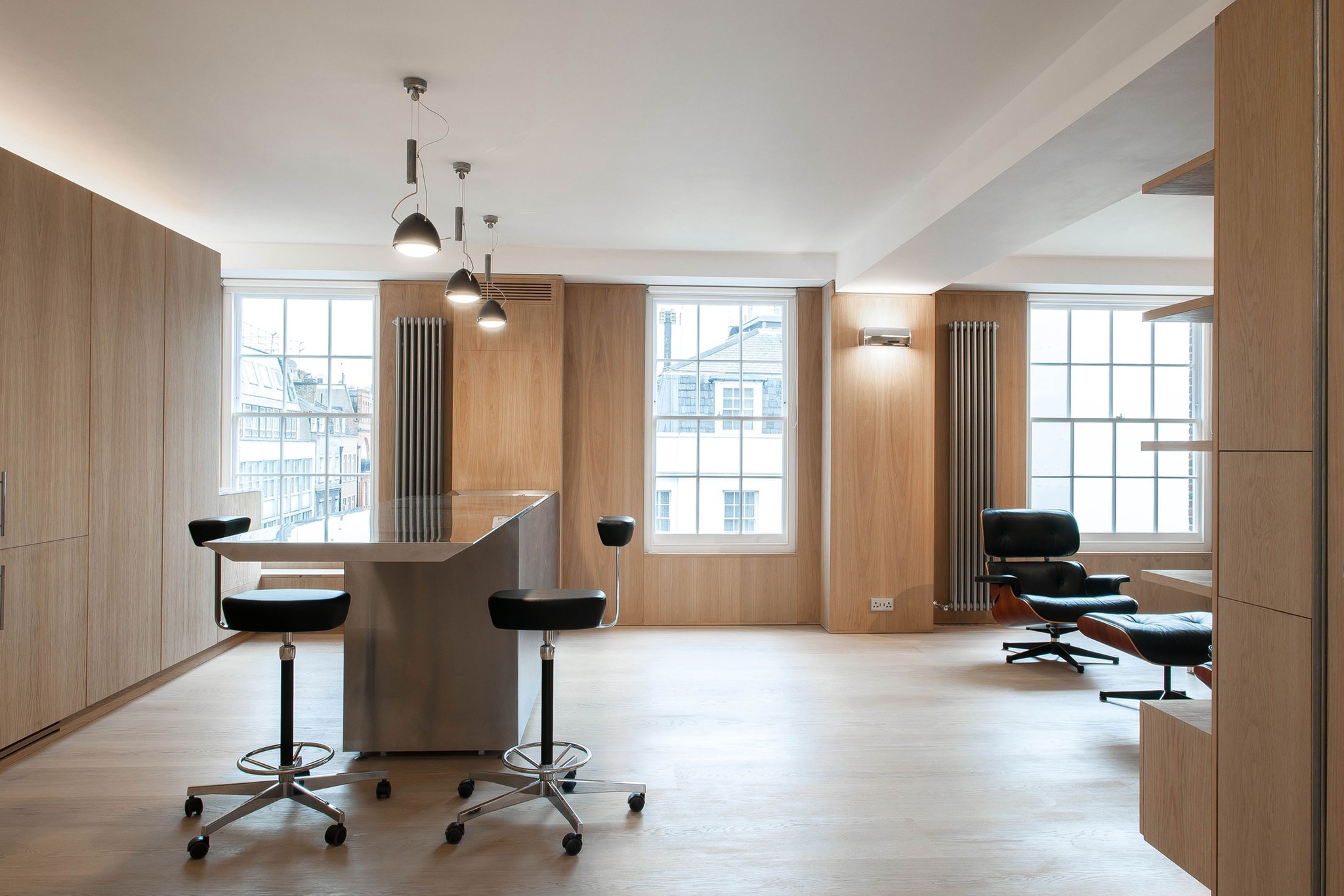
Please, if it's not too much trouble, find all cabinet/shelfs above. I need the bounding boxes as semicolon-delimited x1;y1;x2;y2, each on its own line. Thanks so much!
0;147;261;749
1140;149;1215;890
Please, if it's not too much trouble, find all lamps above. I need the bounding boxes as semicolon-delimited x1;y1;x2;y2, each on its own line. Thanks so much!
859;327;911;347
392;76;507;327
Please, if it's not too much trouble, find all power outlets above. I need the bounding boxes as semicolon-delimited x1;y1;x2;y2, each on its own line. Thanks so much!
870;598;894;611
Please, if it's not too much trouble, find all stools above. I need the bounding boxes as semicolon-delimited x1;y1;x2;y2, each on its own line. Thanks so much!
444;516;647;856
184;516;392;860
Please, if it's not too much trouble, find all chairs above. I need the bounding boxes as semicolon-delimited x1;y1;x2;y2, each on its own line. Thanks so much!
1077;611;1212;700
973;507;1140;674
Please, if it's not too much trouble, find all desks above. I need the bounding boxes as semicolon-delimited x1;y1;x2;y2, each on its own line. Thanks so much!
202;490;560;762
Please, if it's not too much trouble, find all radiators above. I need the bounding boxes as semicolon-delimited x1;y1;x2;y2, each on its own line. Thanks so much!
934;320;999;612
392;317;448;499
